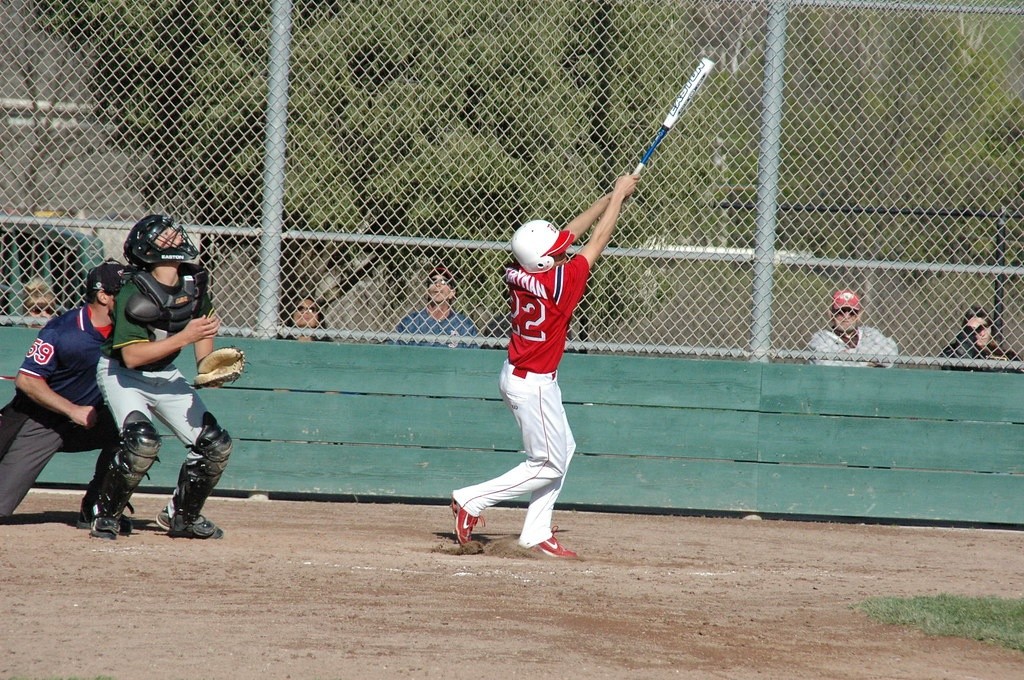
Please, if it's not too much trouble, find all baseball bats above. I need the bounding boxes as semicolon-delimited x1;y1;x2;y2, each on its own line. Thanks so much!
632;56;716;177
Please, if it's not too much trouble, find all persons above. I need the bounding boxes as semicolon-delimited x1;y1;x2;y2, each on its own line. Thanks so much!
452;174;641;557
21;277;55;328
940;308;1021;374
0;256;133;535
90;214;245;540
391;268;478;348
807;289;898;369
482;262;578;352
278;296;334;342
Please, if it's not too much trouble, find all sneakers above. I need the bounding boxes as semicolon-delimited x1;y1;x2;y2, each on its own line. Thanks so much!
452;499;486;545
528;526;576;558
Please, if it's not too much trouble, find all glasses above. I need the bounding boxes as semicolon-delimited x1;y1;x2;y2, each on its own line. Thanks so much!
30;305;55;315
296;307;316;313
836;309;858;316
428;278;449;286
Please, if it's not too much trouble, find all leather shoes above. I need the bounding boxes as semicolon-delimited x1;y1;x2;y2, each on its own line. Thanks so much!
512;368;557;380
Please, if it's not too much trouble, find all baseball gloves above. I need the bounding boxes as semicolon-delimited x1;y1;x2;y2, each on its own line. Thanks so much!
193;343;252;389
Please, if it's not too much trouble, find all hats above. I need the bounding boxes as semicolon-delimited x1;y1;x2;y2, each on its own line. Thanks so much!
832;290;860;310
552;234;575;256
427;268;454;288
86;263;123;294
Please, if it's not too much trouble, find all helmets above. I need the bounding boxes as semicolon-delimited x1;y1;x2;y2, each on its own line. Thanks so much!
122;215;199;272
510;219;570;272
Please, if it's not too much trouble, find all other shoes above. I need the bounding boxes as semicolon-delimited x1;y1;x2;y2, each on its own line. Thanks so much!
156;506;172;530
92;502;116;540
169;515;224;539
76;509;133;536
